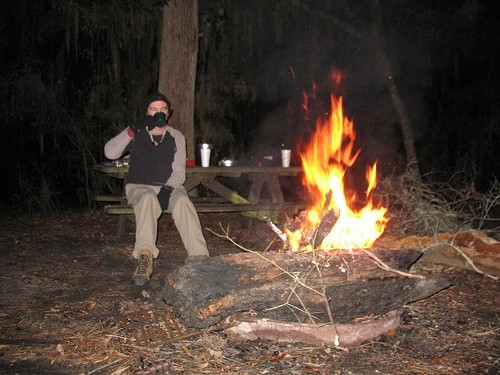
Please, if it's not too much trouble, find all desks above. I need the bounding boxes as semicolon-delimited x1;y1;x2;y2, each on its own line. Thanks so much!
91;164;315;239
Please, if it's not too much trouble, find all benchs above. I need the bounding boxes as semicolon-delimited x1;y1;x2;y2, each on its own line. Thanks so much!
96;196;309;238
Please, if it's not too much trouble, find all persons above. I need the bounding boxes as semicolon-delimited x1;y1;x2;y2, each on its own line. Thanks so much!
104;92;209;282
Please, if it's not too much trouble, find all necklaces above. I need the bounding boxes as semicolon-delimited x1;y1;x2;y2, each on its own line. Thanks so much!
145;125;169;150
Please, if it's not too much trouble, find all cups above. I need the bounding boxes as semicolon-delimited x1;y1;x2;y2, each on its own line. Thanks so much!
281;150;291;168
201;149;211;168
154;112;166;127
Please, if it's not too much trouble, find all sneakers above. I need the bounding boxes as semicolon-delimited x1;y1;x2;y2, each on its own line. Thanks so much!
131;248;153;282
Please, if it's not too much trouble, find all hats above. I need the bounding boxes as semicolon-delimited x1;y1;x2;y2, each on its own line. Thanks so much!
144;92;172;115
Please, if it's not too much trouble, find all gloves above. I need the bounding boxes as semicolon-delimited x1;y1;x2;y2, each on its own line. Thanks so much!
157;186;172;211
130;115;155;133
